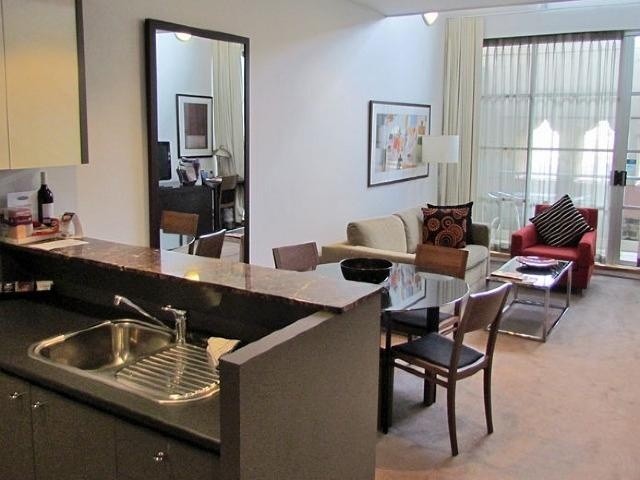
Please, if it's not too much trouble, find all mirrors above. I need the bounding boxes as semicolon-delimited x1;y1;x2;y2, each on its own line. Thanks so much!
144;17;250;261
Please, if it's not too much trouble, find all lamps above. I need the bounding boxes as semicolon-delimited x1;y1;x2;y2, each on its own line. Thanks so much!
421;133;461;205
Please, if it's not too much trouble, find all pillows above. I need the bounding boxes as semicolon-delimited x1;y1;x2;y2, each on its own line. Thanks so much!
420;206;470;248
528;193;594;248
428;201;474;244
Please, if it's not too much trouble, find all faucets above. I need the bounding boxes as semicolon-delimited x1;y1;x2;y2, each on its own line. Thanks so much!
112;294;189;344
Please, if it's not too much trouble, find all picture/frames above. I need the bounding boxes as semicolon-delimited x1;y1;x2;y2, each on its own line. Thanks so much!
367;99;433;188
176;93;214;159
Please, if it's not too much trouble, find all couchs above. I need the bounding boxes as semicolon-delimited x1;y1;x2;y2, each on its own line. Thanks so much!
510;204;600;295
322;201;491;285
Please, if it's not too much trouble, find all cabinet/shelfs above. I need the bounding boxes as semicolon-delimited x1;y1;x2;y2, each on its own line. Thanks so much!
1;0;88;172
0;373;118;479
118;419;221;479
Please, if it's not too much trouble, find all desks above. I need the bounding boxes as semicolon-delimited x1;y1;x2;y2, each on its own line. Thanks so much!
160;231;196;252
310;260;470;435
203;177;244;231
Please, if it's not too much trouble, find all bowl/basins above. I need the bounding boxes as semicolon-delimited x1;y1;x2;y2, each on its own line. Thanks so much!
381;294;393;308
339;257;393;283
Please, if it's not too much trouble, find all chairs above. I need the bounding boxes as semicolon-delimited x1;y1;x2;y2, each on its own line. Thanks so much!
271;239;318;271
161;209;198;255
215;175;238;229
381;244;470;406
383;280;513;458
195;228;227;259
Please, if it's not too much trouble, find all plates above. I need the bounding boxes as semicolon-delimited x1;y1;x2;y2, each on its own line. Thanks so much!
516;255;558;270
515;264;556;276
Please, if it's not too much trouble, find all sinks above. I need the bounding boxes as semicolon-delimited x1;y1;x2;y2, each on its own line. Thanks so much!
28;318;178;373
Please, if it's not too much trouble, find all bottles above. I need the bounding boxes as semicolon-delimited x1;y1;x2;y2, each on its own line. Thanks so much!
37;170;55;224
